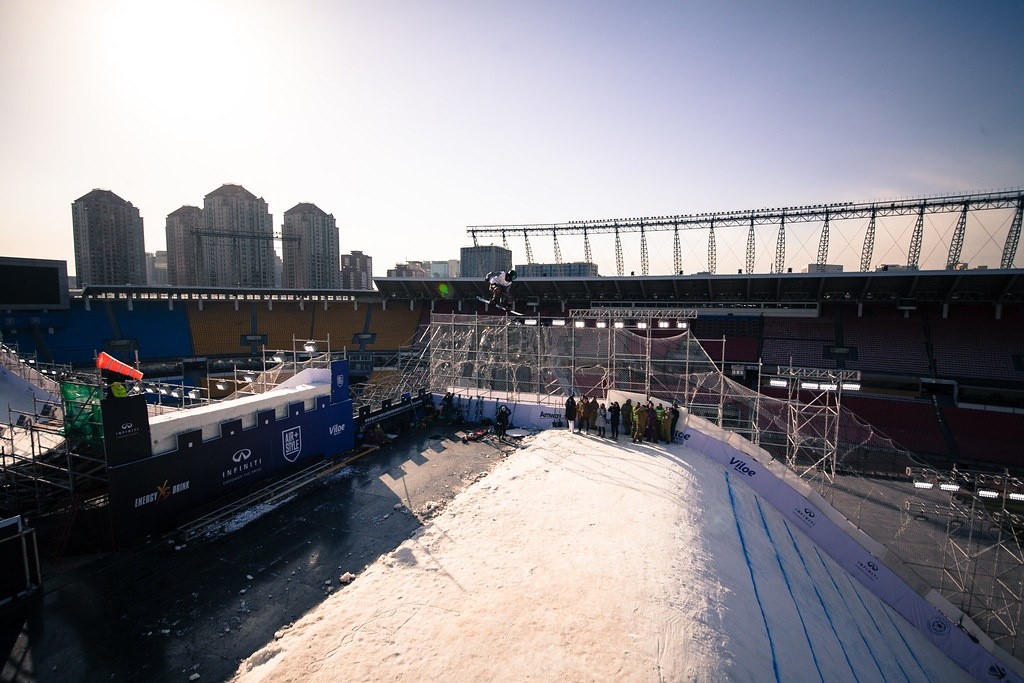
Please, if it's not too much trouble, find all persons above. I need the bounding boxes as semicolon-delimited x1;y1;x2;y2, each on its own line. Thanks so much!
497;406;508;441
486;269;518;313
566;396;680;444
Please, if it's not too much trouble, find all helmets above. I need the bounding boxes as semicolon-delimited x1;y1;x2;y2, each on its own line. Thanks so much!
505;269;518;282
